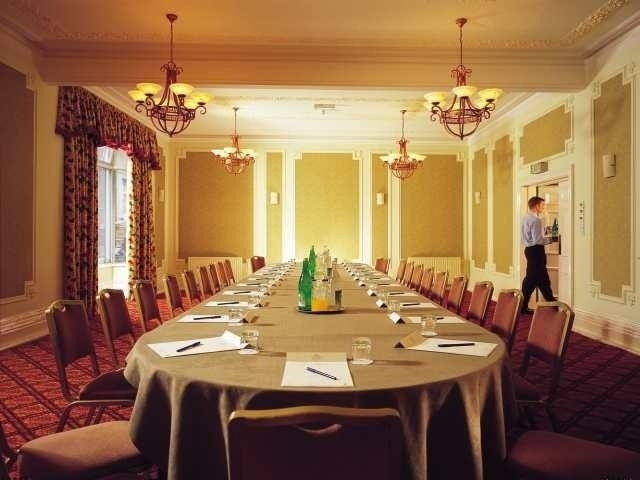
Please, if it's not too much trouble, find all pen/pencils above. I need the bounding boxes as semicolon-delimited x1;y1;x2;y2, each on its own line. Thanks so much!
234;291;251;294
217;301;239;305
193;316;221;320
438;343;475;347
378;284;389;285
437;317;443;320
247;283;259;285
176;342;200;352
403;303;419;306
306;367;336;380
389;293;404;295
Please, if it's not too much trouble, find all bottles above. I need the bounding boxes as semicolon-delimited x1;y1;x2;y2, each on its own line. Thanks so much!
297;245;342;312
551;217;560;241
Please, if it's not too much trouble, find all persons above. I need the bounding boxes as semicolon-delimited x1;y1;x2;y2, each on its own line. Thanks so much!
519;196;558;314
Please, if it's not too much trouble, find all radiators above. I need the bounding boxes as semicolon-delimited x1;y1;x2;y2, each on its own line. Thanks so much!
187;254;243;295
406;255;461;287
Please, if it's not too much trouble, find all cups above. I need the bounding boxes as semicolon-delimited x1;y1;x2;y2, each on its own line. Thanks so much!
227;309;244;326
248;259;296;310
351;336;371;364
239;330;259;354
420;314;438;337
350;265;390;303
387;298;401;314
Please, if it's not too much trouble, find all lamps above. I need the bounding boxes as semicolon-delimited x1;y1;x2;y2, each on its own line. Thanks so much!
212;107;259;177
380;109;429;182
126;12;216;141
422;17;503;139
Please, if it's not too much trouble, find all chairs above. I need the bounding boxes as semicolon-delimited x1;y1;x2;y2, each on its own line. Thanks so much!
0;417;151;479
496;298;579;427
510;428;637;480
133;252;504;480
46;299;135;421
94;288;137;365
131;279;163;334
491;289;522;362
